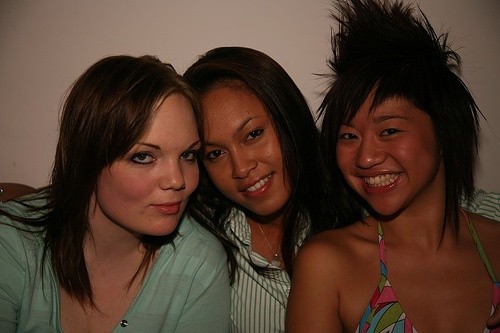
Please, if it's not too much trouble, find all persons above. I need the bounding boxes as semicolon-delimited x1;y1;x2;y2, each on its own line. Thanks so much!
0;46;370;333
284;0;500;333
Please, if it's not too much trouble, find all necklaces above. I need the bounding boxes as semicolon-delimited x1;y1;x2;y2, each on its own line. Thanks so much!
255;218;283;257
